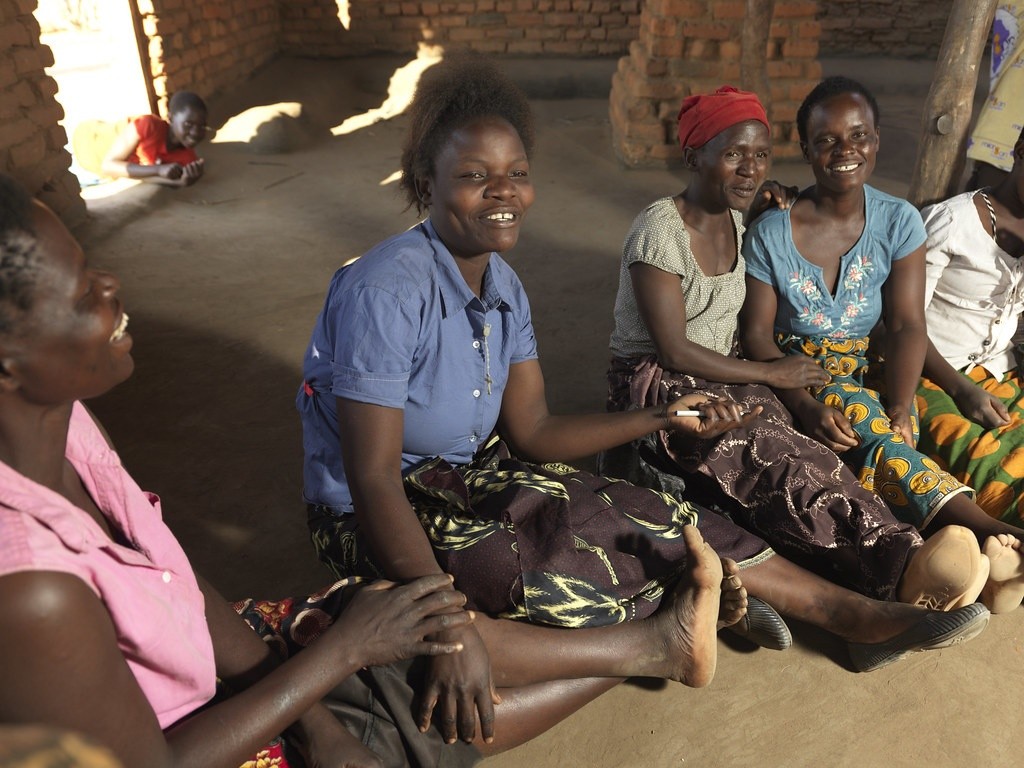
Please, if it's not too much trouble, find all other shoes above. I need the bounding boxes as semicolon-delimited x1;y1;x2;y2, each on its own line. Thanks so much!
847;603;990;672
902;525;981;611
744;594;792;651
940;553;990;612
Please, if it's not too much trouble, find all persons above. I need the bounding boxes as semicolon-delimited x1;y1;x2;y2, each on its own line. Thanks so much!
0;185;748;768
298;57;989;747
608;86;991;644
64;90;208;188
915;127;1024;529
737;78;1024;615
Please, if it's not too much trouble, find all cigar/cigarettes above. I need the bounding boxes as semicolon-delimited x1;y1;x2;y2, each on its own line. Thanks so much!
675;410;748;417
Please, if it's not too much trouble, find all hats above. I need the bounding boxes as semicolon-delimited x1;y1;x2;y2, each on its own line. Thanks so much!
676;85;771;154
